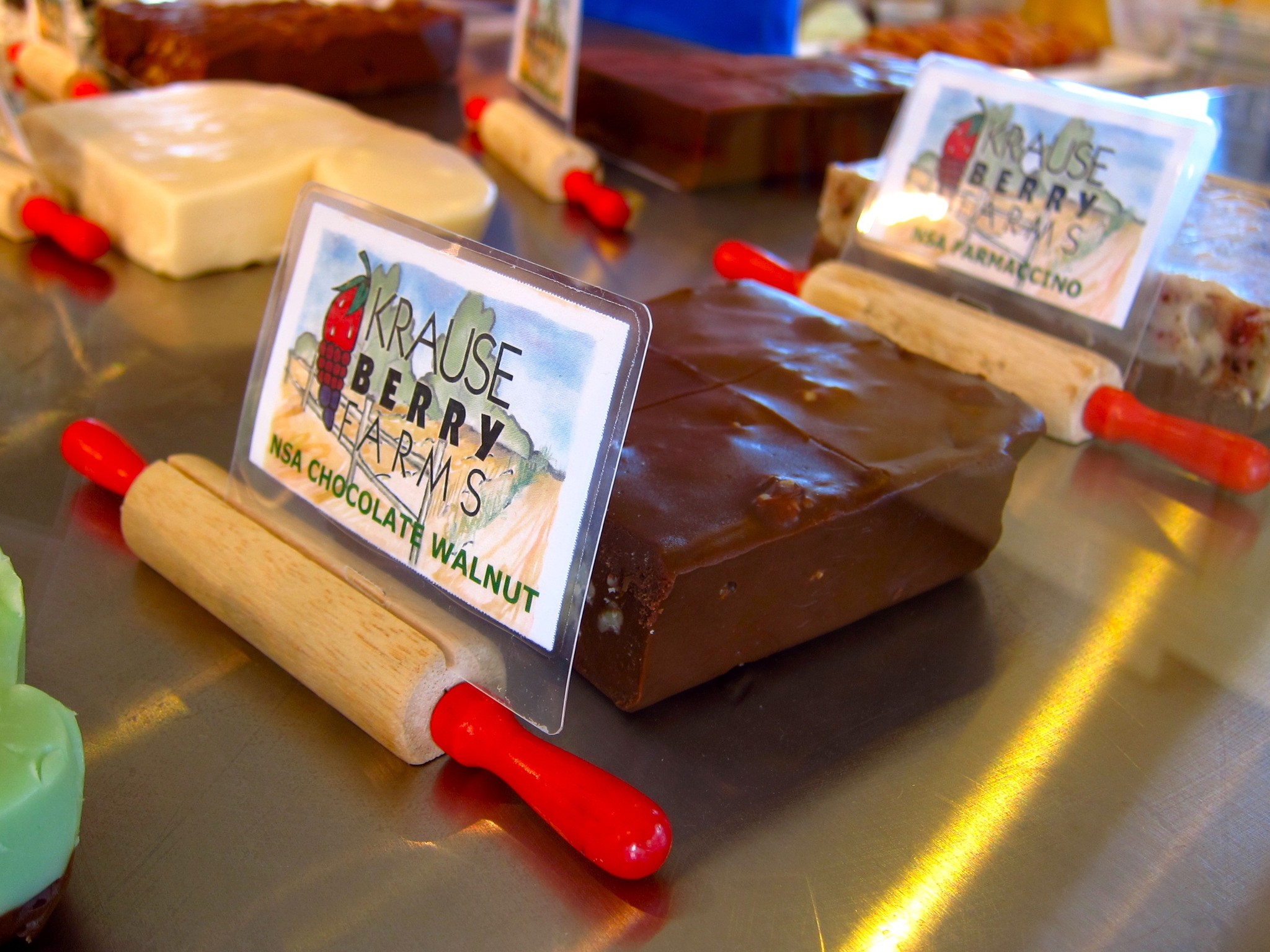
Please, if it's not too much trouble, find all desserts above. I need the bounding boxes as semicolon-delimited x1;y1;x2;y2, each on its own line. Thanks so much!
94;1;464;93
810;158;1268;407
571;277;1048;715
21;81;502;280
575;24;906;192
